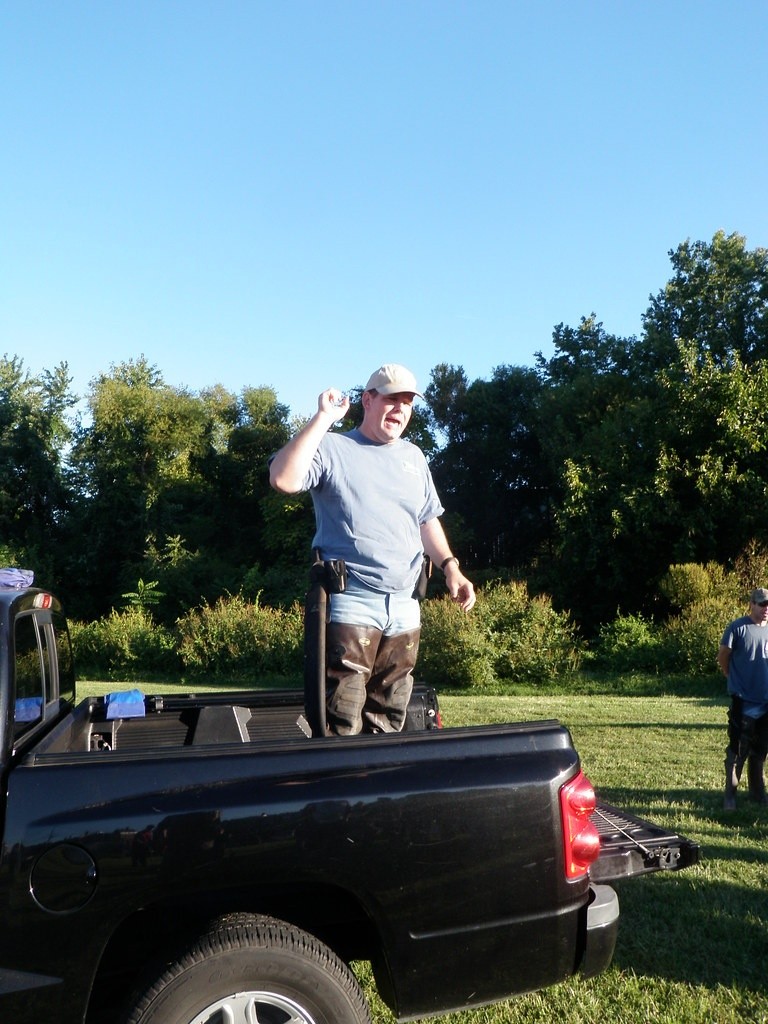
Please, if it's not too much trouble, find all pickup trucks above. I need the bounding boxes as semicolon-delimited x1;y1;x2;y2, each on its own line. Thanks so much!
0;583;701;1024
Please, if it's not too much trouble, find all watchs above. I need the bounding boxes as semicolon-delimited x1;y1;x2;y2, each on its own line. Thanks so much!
440;557;459;572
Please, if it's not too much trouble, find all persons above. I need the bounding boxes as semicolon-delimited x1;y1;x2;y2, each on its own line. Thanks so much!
271;364;477;737
715;587;768;813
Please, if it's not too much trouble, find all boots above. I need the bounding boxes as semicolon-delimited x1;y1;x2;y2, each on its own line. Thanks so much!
325;622;383;737
747;763;768;803
723;760;743;813
362;626;421;733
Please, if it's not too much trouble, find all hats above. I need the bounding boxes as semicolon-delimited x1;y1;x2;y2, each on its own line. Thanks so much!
751;589;768;603
364;363;424;400
